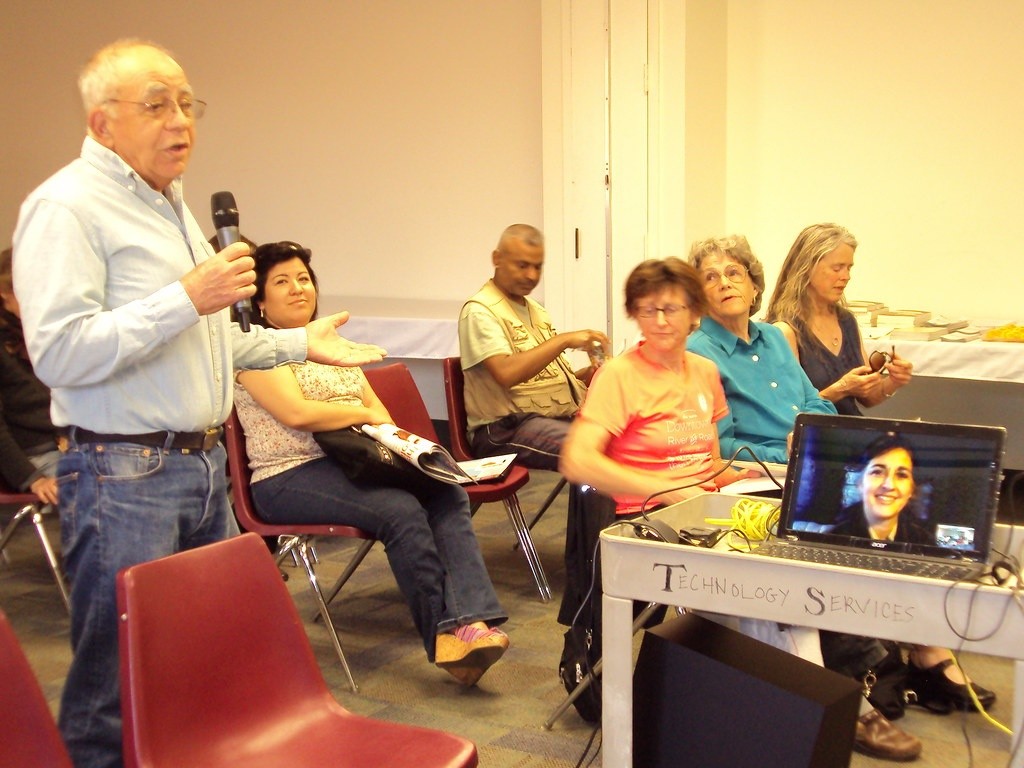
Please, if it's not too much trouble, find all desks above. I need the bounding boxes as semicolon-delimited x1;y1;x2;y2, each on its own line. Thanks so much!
336;315;462;420
861;337;1024;472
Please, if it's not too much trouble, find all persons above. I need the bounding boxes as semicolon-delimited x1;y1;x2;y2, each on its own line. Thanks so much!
234;241;511;687
684;237;952;720
558;258;923;763
765;224;997;714
823;435;935;545
458;224;618;580
208;233;289;581
11;40;387;768
0;249;58;507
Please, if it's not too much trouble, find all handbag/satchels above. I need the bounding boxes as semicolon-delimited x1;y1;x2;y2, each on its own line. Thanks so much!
311;424;425;489
558;625;605;723
830;663;905;720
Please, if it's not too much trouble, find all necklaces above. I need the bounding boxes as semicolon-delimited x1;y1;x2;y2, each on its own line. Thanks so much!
832;338;837;346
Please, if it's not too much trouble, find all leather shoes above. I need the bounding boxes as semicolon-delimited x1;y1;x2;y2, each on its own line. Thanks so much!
907;653;996;705
853;695;922;760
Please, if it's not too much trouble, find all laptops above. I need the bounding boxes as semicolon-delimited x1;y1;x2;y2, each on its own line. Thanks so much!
749;414;1007;584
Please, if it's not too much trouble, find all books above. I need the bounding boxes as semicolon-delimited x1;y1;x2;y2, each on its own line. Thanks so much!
844;300;997;342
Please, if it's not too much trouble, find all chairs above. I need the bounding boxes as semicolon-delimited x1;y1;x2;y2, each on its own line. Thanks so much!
0;356;659;768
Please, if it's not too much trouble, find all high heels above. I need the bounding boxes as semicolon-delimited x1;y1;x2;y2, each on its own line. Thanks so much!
435;625;507;667
446;628;507;686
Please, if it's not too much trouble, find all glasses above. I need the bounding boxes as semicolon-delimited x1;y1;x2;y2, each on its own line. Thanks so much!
701;263;751;288
637;305;697;318
258;241;305;261
865;345;895;374
109;97;207;119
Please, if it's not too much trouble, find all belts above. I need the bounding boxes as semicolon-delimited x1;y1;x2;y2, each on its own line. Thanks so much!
54;424;222;450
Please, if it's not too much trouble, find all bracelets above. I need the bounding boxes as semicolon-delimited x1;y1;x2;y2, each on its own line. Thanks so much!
883;391;897;398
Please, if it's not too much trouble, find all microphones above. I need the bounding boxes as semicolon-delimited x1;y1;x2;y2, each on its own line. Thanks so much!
211;190;254;333
